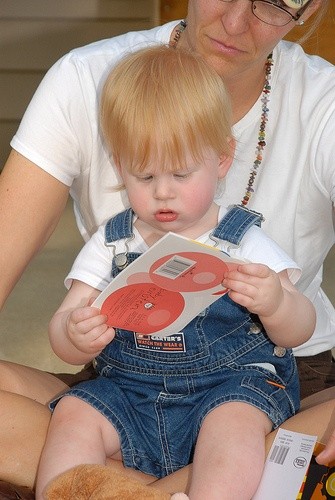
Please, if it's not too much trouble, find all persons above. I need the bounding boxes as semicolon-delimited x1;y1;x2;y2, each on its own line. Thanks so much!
0;0;335;500
35;45;317;500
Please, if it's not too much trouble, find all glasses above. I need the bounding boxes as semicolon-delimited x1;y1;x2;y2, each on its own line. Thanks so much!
250;0;315;28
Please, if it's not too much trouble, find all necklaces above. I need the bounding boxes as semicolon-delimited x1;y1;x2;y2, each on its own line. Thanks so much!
172;20;275;209
